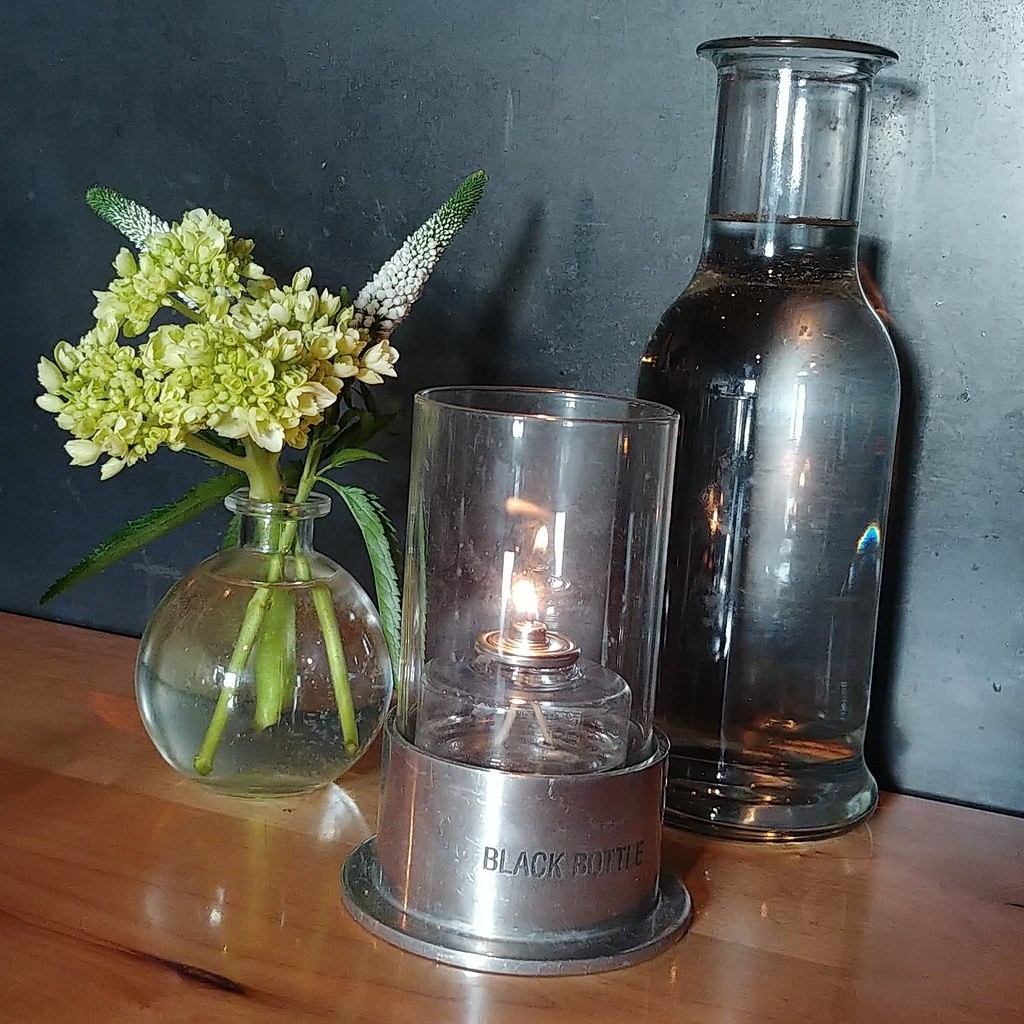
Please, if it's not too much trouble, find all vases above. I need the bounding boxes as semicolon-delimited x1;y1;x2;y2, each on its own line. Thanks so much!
134;489;395;799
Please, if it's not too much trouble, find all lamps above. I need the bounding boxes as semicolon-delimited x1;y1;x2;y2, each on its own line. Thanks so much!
341;386;693;974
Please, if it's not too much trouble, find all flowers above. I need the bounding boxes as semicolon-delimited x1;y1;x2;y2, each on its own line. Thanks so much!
39;173;489;775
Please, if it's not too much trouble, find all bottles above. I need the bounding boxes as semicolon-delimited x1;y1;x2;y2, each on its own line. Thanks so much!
639;36;904;842
